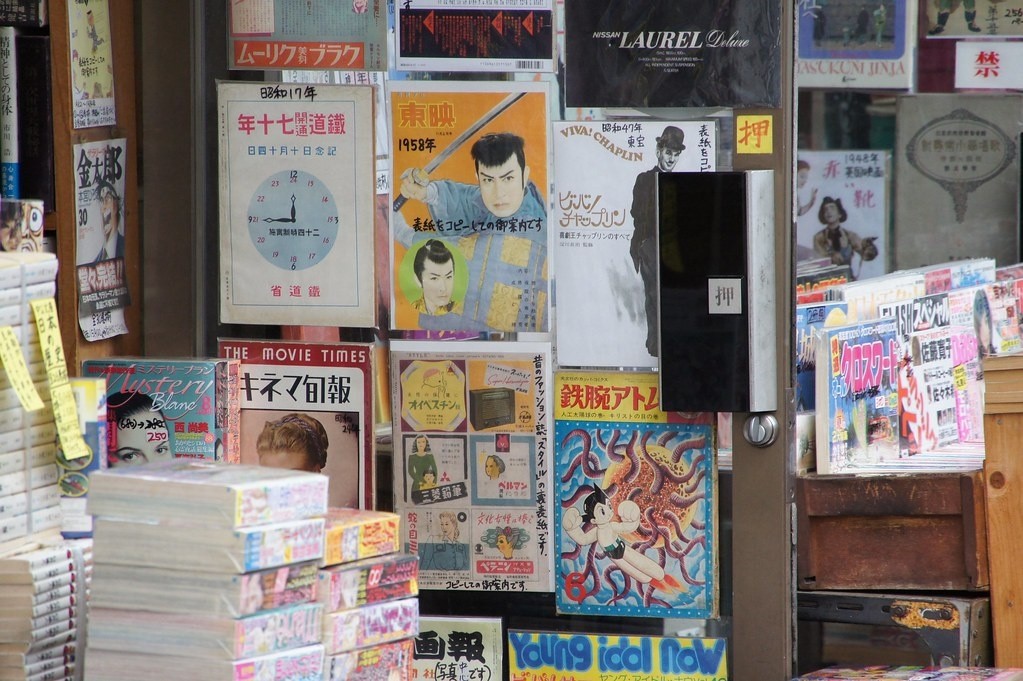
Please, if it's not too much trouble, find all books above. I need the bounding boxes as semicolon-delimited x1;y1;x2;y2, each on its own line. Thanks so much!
383;0;778;681
58;342;419;681
0;0;91;681
794;254;1022;477
794;0;1020;282
219;76;379;330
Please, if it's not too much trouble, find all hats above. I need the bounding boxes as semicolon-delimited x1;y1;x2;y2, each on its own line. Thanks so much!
655;126;685;150
